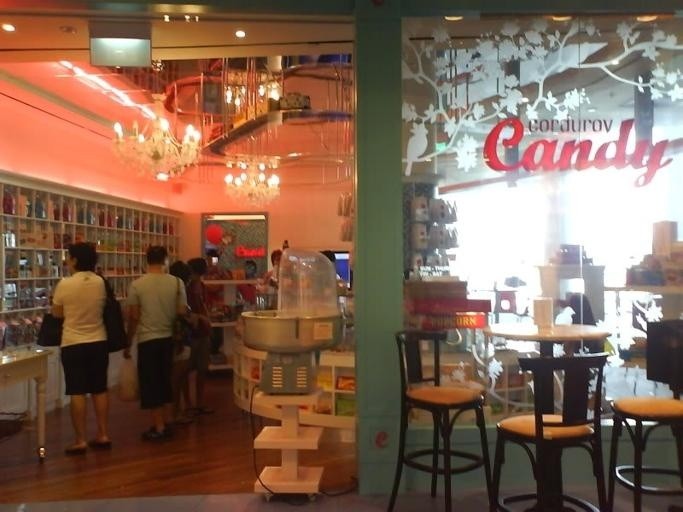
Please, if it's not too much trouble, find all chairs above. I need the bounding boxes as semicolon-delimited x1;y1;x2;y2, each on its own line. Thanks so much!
490;352;606;512
389;330;494;509
608;320;682;511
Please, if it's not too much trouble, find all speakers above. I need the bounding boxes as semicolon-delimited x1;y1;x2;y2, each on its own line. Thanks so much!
87;22;153;69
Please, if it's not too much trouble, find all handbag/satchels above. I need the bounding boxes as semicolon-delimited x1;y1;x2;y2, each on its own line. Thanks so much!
172;321;192;374
38;313;64;347
97;274;129;353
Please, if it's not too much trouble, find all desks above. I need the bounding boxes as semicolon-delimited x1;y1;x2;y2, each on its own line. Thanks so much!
1;347;52;460
484;323;612;418
537;260;604;329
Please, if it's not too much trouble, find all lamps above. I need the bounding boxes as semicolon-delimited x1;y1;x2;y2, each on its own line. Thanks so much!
111;61;200;178
224;52;279;105
224;139;281;204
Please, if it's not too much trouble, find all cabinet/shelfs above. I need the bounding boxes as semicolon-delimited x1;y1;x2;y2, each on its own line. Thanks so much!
0;344;60;421
61;337;136;406
610;223;682;371
250;387;325;501
0;168;184;346
232;345;529;428
198;275;264;370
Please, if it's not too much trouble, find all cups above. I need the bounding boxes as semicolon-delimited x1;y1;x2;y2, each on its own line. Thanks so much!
537;326;553;335
533;298;553;328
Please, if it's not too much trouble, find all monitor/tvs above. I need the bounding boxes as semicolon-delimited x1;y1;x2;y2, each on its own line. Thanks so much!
332;251;352;291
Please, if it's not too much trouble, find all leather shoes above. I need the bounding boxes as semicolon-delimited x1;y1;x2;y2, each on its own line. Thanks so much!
66;443;87;453
88;436;112;449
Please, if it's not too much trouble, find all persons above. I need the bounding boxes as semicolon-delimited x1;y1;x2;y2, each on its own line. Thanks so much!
259;250;298;310
237;258;259;306
50;240;115;455
118;247;192;442
167;259;200;428
185;256;226;416
203;244;229;316
310;249;351;306
